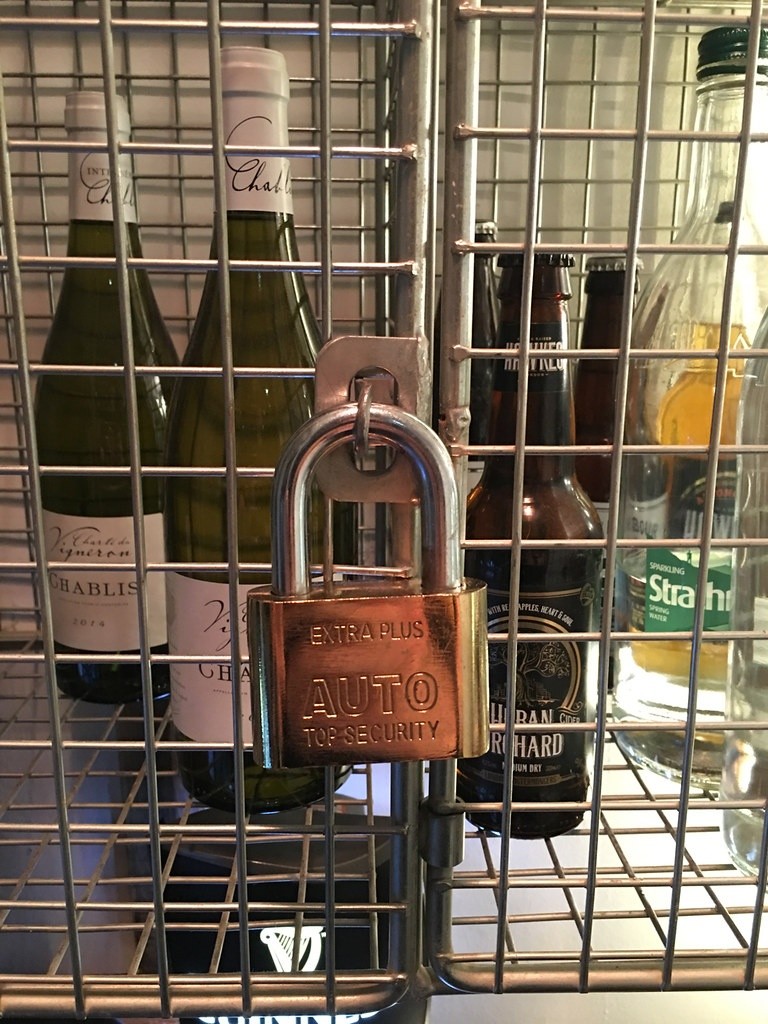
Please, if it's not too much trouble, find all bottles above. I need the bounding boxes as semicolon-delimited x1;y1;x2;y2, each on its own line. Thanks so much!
34;92;181;700
163;45;360;804
436;204;768;880
610;25;768;791
